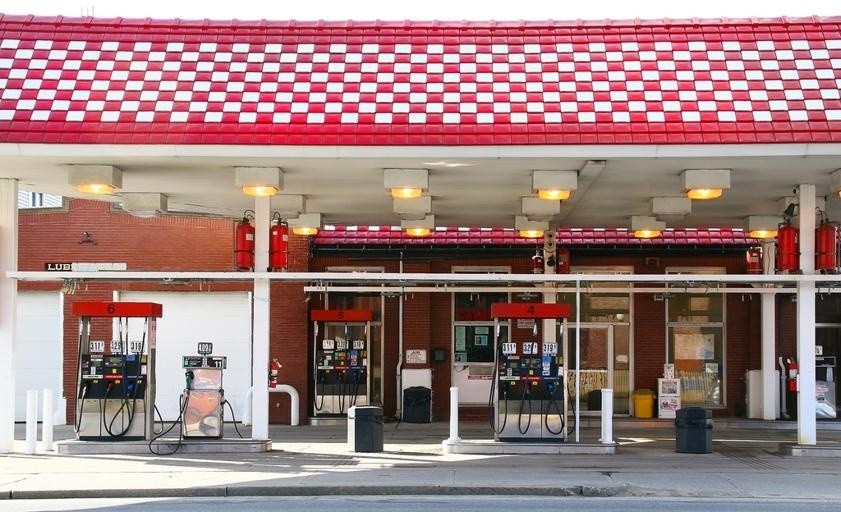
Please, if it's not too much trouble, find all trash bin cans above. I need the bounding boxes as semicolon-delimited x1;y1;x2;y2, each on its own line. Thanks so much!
674;407;713;454
588;390;601;410
403;386;431;423
632;388;657;419
347;405;384;453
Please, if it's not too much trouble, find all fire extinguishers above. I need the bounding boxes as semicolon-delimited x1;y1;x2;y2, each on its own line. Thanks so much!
556;246;569;274
269;358;283;388
814;206;837;271
746;243;764;274
269;211;289;272
778;204;798;272
233;209;255;271
789;356;798;392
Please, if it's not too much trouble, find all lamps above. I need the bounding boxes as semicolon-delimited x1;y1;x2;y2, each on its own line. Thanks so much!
648;197;692;221
521;196;561;221
744;215;778;238
401;215;435;236
384;168;429;199
629;216;666;238
234;168;284;196
531;170;577;199
393;196;431;220
680;170;731;199
121;193;167;218
271;196;306;219
514;215;549;237
66;164;123;196
288;213;323;234
